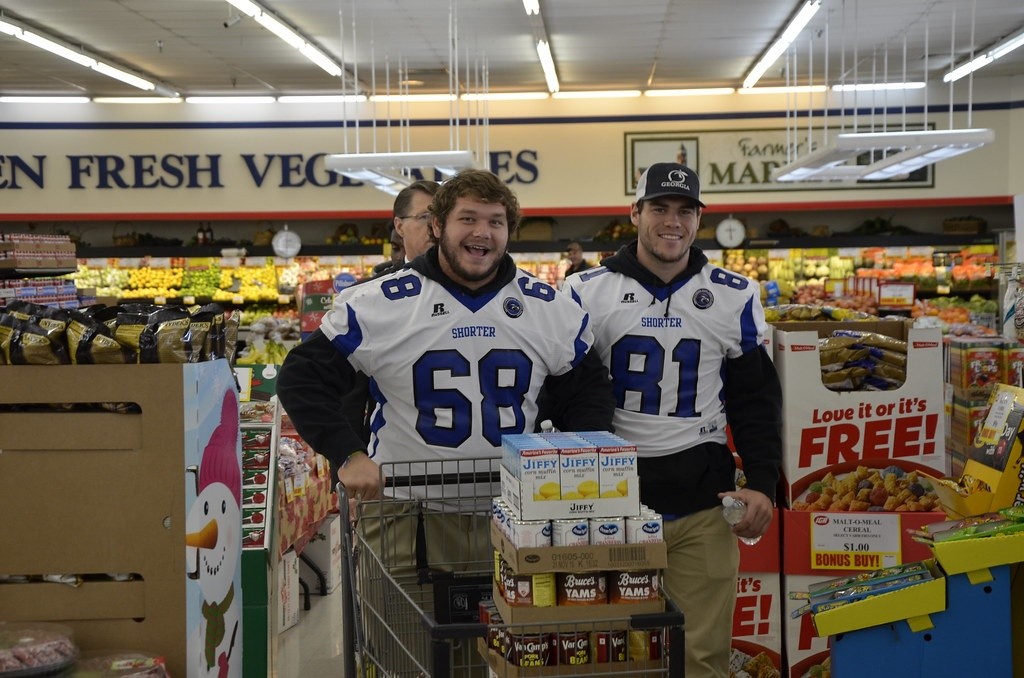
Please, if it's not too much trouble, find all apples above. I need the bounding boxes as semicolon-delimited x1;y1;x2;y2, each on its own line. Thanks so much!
238;302;299;326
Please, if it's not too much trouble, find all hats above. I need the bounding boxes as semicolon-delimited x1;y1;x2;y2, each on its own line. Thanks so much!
565;242;581;252
637;163;706;208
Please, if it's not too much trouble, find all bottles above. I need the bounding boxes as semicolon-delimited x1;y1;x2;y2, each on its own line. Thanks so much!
722;495;761;545
540;420;562;433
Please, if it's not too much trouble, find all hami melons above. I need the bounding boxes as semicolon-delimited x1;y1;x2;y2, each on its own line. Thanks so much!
724;255;768;280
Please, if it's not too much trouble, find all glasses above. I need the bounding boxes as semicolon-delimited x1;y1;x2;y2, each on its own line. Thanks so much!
397;212;436;223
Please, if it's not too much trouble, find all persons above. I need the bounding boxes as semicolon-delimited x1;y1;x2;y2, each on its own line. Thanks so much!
371;222;407;278
275;169;617;678
329;180;440;522
565;243;596;276
563;163;783;678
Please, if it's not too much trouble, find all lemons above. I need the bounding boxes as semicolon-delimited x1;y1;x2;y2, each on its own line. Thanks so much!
119;268;280;302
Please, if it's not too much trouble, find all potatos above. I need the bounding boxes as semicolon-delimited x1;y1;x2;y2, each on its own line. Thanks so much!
792;285;876;315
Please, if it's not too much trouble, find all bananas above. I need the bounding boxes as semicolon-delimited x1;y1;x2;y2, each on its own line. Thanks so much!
236;337;300;365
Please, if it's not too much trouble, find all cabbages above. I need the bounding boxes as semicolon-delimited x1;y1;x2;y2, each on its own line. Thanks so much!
769;261;831;287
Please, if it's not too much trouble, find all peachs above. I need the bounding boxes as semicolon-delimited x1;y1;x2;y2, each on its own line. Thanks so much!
521;264;556;289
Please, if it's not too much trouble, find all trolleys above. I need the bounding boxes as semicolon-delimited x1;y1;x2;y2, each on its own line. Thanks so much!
336;456;687;678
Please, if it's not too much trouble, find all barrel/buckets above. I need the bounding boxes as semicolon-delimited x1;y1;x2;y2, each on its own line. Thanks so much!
481;496;664;668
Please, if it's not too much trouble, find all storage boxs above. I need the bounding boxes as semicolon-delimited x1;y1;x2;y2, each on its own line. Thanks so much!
476;464;666;677
0;241;96;309
0;359;242;678
772;320;1024;678
277;509;343;632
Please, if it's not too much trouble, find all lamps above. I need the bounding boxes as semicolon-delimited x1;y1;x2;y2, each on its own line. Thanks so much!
772;0;994;181
325;0;490;196
0;0;1024;103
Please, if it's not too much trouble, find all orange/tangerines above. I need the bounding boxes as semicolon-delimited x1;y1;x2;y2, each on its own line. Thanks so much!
912;303;993;334
